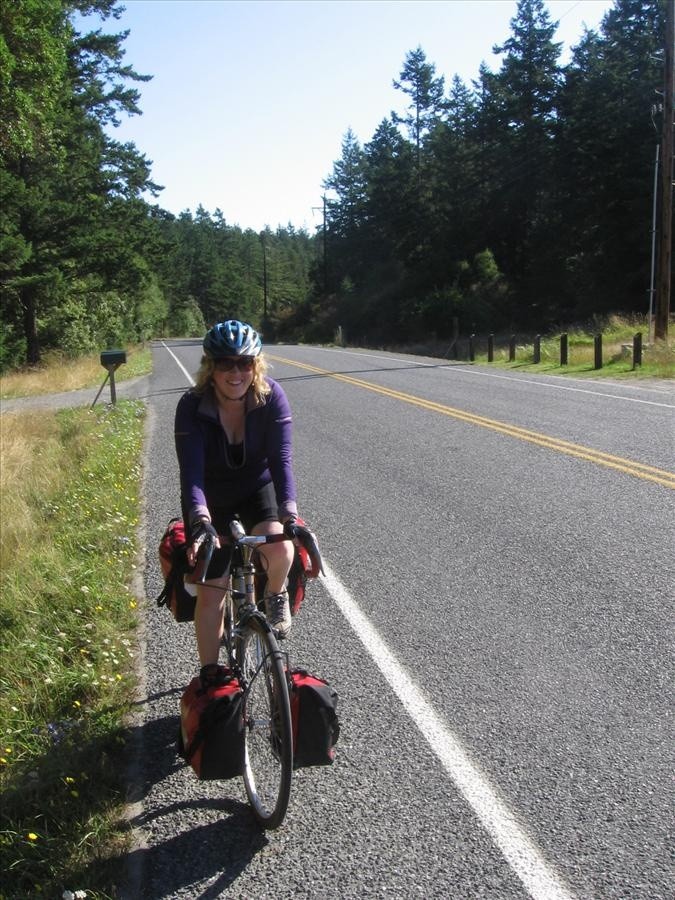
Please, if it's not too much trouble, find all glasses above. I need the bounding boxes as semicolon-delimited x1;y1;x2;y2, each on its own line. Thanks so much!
213;357;256;371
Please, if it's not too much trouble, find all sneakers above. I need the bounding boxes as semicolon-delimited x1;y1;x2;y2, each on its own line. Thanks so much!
263;580;291;638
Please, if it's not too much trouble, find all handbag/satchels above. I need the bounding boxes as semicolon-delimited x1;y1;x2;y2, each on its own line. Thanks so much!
157;517;198;623
254;516;320;614
176;664;245;780
272;666;340;771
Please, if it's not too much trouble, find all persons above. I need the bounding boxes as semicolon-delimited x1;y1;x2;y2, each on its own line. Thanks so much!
174;321;311;691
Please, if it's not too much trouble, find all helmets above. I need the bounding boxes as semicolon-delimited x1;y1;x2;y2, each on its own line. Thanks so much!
203;320;263;357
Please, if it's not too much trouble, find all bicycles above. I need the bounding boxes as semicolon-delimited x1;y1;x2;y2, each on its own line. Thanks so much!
194;514;328;831
